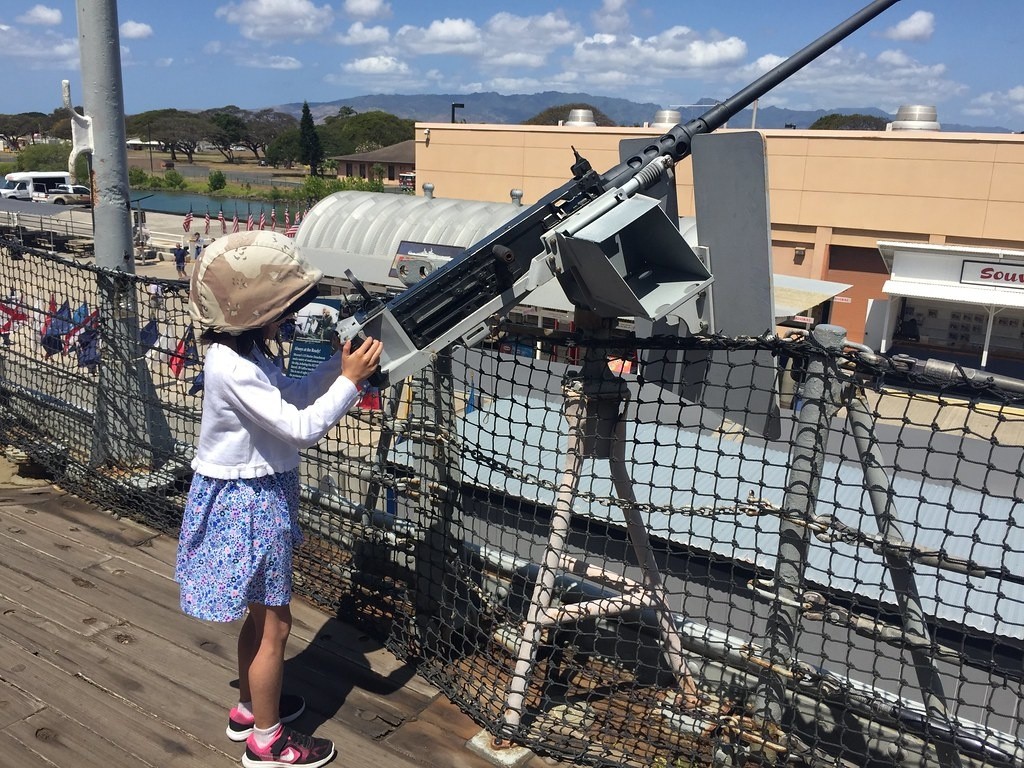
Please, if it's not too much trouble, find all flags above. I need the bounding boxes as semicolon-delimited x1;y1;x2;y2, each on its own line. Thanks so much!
204;204;211;234
232;207;240;233
217;203;227;234
183;201;193;232
245;197;310;238
0;288;200;380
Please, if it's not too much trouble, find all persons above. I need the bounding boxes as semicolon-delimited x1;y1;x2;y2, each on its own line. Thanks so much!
173;231;384;768
896;317;920;342
149;233;205;320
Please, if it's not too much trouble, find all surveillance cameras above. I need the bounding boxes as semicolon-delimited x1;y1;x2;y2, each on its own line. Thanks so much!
424;130;428;135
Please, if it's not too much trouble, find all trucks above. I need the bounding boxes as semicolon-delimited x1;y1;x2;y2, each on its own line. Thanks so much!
0;171;71;201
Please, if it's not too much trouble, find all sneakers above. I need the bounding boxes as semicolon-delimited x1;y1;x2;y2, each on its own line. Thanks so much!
225;692;306;742
242;724;335;768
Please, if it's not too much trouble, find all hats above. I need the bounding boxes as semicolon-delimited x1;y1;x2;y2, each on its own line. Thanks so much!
188;230;323;334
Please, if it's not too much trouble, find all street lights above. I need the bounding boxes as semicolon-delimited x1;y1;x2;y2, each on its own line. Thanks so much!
148;120;154;171
452;102;465;123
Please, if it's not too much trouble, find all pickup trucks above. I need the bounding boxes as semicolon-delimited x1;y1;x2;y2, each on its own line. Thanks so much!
31;180;92;205
